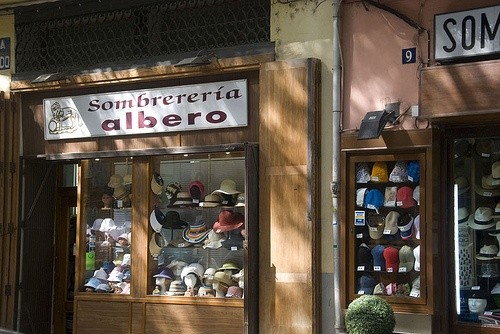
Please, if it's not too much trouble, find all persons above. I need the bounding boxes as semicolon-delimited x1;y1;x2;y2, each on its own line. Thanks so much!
468;290;487;314
187;181;204;207
100;190;113;209
108;175;130;201
165;182;182;208
211;180;241;205
117;234;128;246
152;262;244;299
85;270;128;294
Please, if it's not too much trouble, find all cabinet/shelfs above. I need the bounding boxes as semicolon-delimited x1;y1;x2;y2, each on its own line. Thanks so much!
448;137;500;328
72;150;258;334
341;127;438;314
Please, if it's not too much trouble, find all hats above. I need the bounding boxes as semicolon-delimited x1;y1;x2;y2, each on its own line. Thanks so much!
450;141;499;299
355;161;419;298
83;172;245;299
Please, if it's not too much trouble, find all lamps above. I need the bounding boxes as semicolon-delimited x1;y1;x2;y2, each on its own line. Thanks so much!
357;99;402;141
